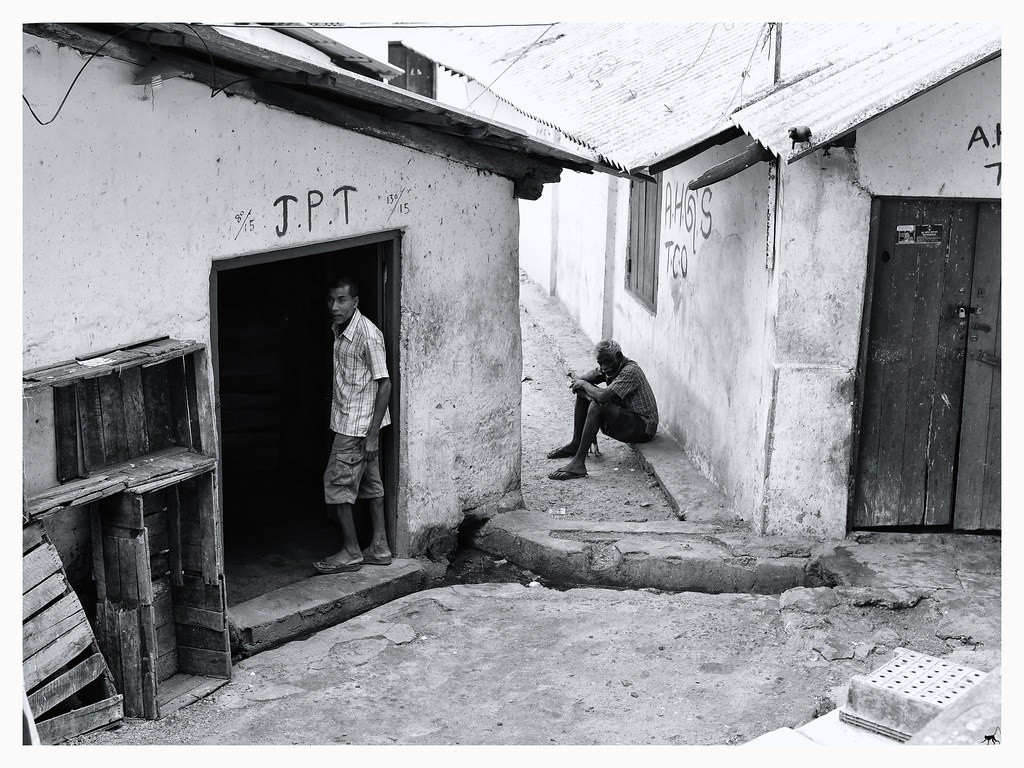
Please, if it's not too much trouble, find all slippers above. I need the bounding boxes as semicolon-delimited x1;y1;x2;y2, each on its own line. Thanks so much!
547;448;577;459
314;555;360;574
362;550;392;565
549;468;587;479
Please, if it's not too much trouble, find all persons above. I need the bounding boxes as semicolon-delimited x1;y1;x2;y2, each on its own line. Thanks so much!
546;339;659;480
310;277;392;573
